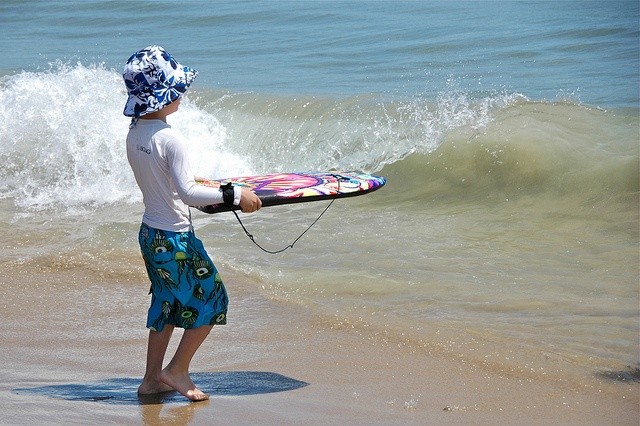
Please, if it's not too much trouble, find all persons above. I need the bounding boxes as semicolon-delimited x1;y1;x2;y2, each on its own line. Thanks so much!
120;43;265;403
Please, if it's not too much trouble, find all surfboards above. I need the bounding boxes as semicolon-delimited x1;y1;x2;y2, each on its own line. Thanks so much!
193;172;386;214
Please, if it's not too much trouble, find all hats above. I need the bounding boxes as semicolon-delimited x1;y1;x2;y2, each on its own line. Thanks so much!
122;44;199;129
